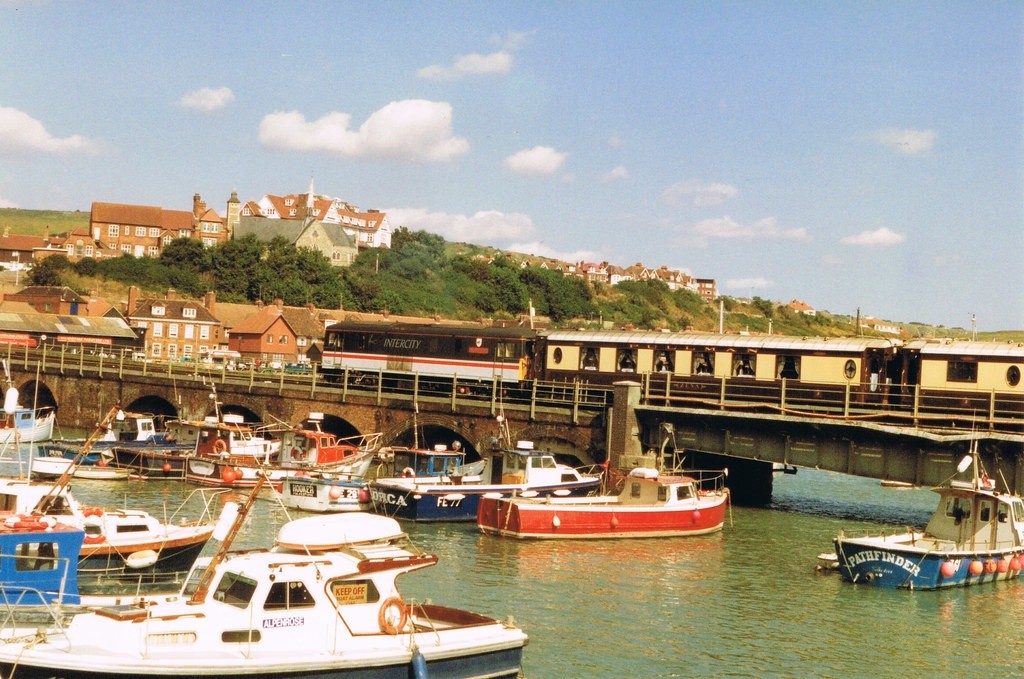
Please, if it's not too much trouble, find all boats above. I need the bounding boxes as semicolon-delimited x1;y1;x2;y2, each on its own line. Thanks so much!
0;404;233;586
0;359;55;443
832;408;1024;590
280;474;378;511
37;433;176;464
31;456;135;480
477;423;733;540
368;344;604;521
0;360;119;518
112;439;281;479
0;477;528;679
185;359;383;488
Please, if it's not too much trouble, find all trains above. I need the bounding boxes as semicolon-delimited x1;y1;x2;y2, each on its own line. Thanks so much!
321;314;1024;431
